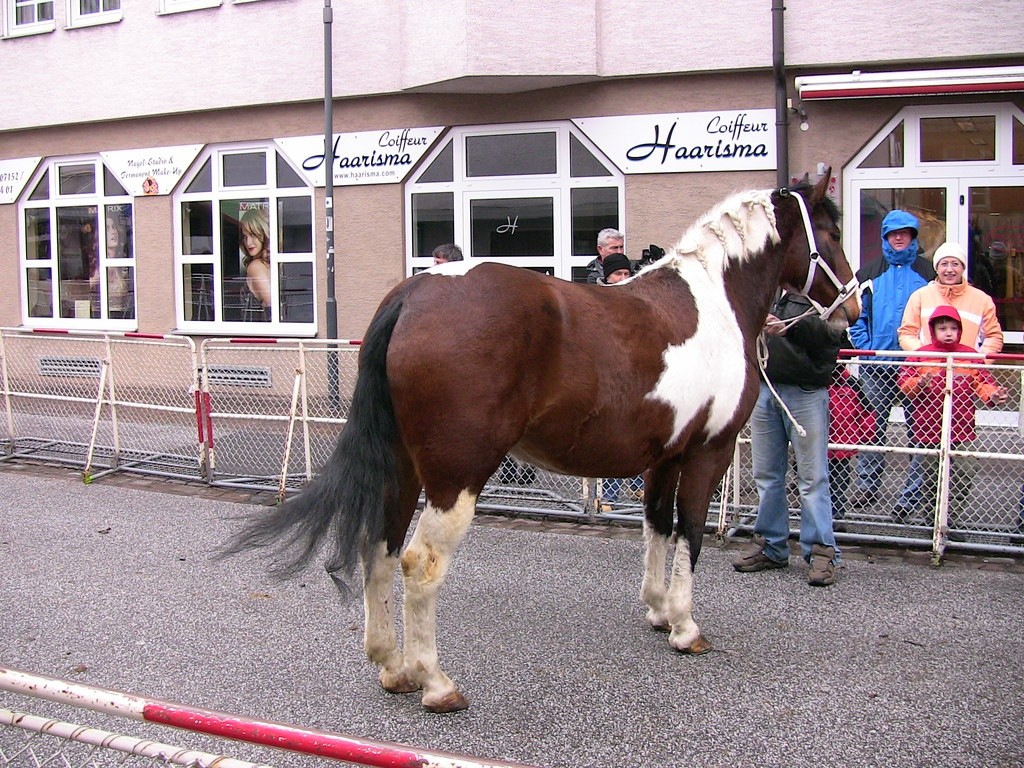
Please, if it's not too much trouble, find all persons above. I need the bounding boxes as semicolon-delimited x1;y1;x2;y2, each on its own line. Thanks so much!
500;456;536;483
431;243;464;267
826;331;876;533
595;253;633;286
891;307;1008;542
586;228;661;510
89;213;129;311
893;242;1003;517
848;209;937;516
238;209;271;322
732;284;842;587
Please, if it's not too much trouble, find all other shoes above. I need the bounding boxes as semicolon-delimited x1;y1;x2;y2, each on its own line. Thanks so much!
1010;518;1024;546
849;489;878;508
929;522;965;542
891;504;912;520
594;497;613;511
629;488;645;501
832;517;848;532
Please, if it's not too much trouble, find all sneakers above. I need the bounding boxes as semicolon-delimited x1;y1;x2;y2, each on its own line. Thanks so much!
807;544;835;586
733;532;789;572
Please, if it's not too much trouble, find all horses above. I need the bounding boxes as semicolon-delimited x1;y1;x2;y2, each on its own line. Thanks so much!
205;165;861;713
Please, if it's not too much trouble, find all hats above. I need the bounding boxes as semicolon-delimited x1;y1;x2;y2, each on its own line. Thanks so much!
990;241;1008;260
601;252;632;282
933;242;967;271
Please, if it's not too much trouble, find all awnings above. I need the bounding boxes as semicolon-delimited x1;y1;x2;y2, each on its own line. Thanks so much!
793;65;1024;100
